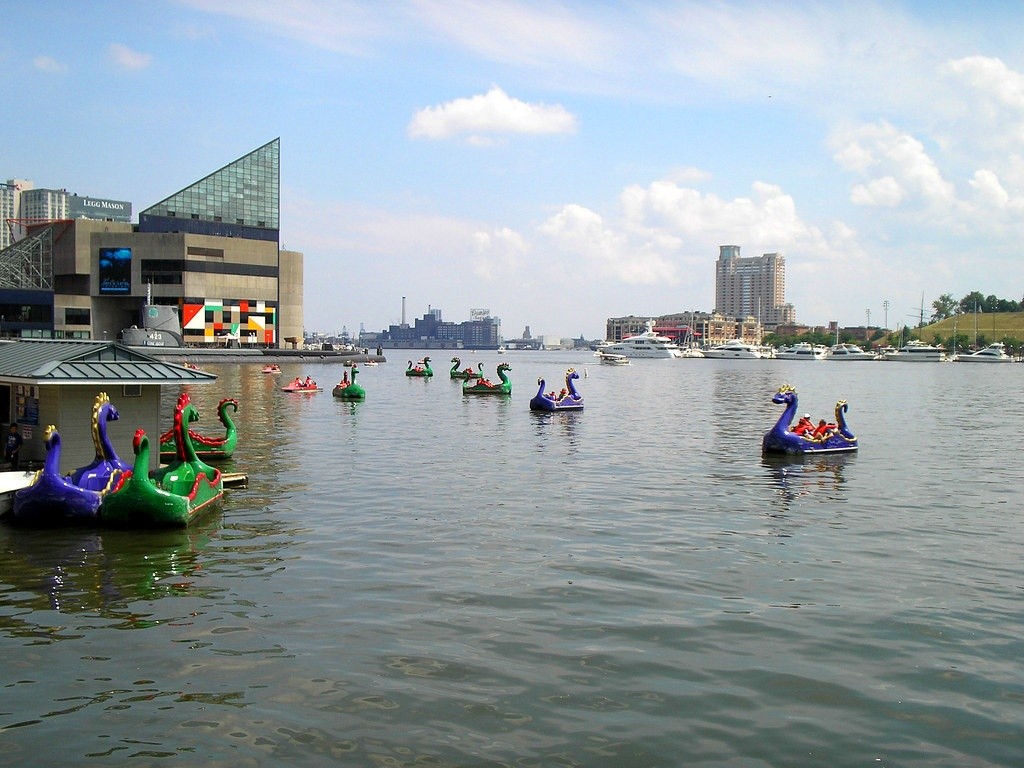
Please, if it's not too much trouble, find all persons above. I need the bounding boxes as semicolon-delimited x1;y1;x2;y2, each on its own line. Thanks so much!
549;387;567;401
4;423;23;471
478;377;493;388
794;413;838;444
266;363;473;389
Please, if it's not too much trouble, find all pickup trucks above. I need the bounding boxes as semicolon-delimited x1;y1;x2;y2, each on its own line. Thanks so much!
813;344;829;355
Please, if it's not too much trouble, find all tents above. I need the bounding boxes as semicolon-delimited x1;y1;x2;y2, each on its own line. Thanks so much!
217;333;241;349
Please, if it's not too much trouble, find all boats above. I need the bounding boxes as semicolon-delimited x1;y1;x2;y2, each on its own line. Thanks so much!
98;391;224;528
343;360;352;366
183;363;199;371
599;353;630;366
884;339;947;363
450;357;485;378
160;398;239;462
599;332;682;359
496;346;505;354
762;383;859;456
364;361;378;366
700;340;764;359
405;356;434;377
530;367;585;412
462;362;512;396
12;391;134;527
954;342;1016;363
332;362;366;400
822;343;878;360
774;343;816;360
281;379;321;392
262;365;282;375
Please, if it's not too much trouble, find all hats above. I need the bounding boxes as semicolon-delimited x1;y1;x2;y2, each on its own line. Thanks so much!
804;413;810;418
